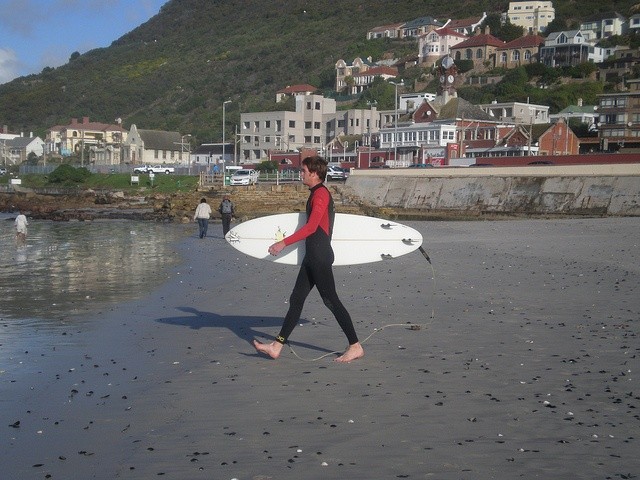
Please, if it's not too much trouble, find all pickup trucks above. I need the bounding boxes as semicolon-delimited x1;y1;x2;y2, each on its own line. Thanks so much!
148;164;175;175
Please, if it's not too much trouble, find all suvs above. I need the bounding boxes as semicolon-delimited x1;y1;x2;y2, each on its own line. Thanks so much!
134;164;151;173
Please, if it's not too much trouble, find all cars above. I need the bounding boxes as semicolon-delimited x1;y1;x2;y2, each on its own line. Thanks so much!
0;168;7;176
528;160;554;166
325;166;349;181
469;163;496;168
369;165;390;169
408;164;432;168
230;168;258;186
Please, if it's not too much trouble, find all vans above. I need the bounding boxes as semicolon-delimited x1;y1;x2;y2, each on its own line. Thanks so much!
225;166;243;181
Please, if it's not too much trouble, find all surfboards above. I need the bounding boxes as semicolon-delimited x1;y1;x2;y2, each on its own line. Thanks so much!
225;212;424;266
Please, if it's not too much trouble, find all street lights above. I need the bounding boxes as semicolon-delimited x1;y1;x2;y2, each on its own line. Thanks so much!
389;81;405;168
180;134;192;167
222;100;234;187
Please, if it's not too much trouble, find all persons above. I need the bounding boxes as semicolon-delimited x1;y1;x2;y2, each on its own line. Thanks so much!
193;198;212;239
14;210;30;239
218;195;234;239
252;156;364;363
